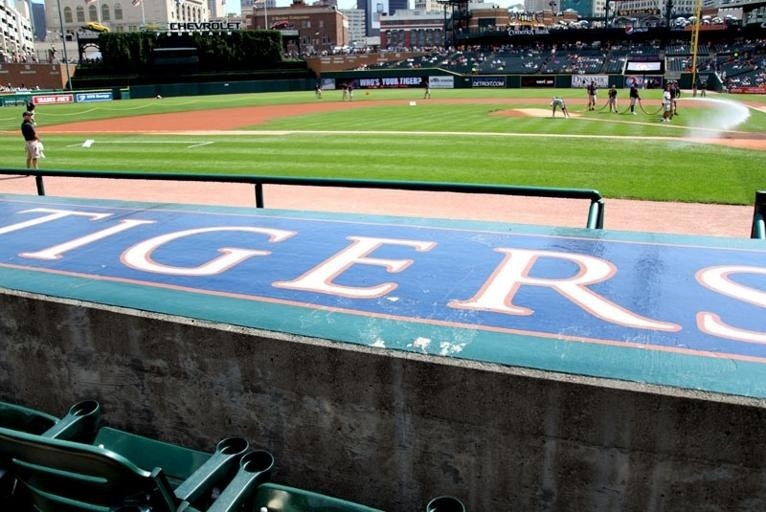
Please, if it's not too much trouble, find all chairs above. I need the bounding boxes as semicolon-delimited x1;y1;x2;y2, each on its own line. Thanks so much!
0;399;251;511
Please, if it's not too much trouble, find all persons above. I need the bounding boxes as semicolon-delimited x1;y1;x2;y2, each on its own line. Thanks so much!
21;102;45;169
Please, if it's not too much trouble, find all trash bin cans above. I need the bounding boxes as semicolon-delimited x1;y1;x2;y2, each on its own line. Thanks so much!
119;88;130;99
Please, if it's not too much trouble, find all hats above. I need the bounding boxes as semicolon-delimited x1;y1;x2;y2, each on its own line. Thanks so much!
22;111;32;116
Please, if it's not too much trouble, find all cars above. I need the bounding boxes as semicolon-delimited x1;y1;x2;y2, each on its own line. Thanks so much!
80;21;111;34
268;20;288;31
